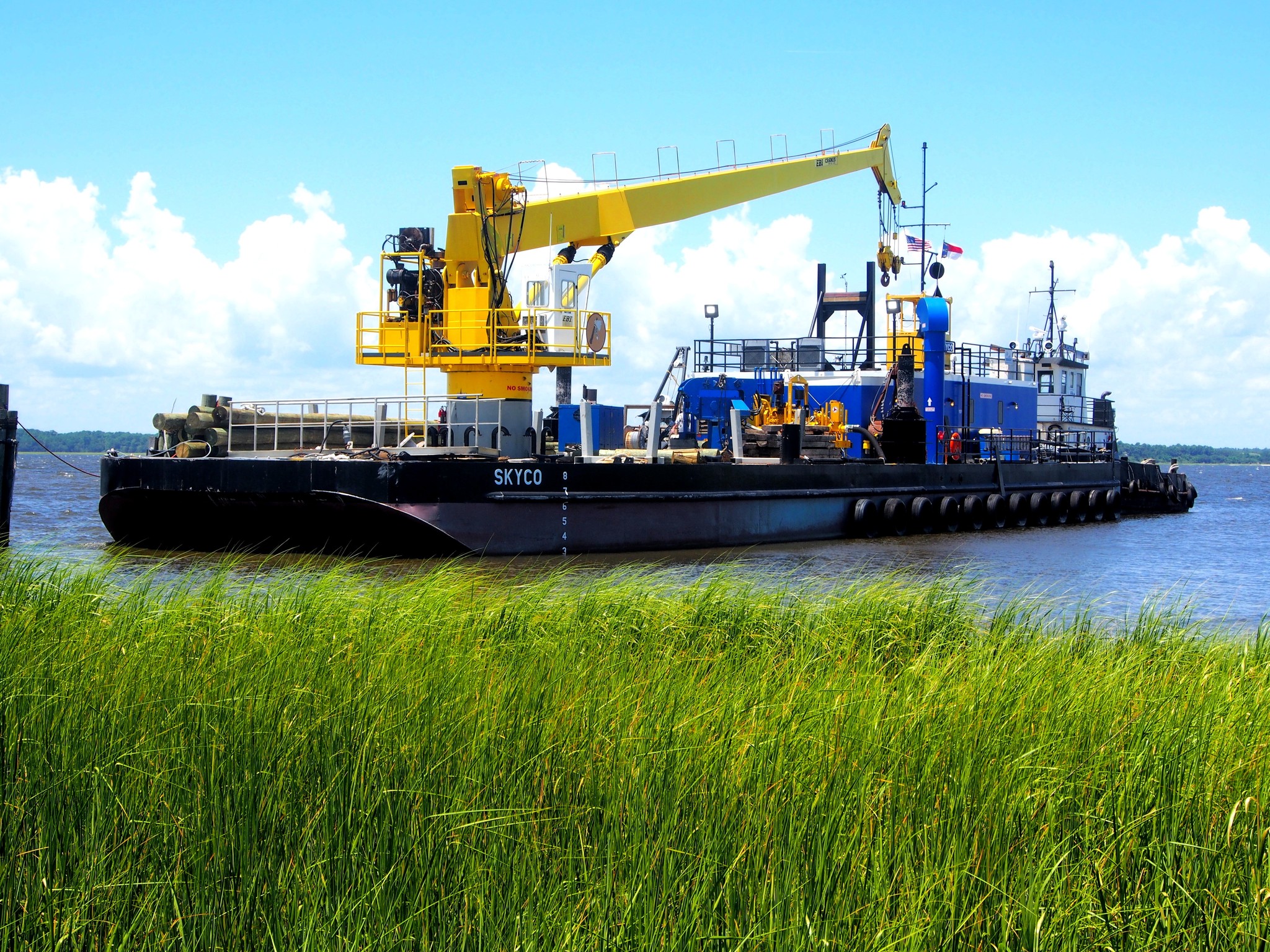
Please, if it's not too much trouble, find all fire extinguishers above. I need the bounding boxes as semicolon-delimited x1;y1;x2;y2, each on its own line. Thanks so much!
437;405;447;434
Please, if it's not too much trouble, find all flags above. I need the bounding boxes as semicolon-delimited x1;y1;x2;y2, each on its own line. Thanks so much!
906;235;932;251
941;242;963;260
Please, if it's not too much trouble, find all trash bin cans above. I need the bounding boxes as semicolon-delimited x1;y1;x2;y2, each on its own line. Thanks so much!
779;424;800;464
1121;457;1129;461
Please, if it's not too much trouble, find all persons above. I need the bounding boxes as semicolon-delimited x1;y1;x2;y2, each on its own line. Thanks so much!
672;413;691;432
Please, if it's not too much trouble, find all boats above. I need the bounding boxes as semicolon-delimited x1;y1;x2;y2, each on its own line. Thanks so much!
99;125;1202;560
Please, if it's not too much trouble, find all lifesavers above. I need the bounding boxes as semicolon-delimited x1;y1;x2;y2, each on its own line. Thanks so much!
1070;490;1089;523
963;494;985;532
1187;483;1198;508
939;496;961;534
911;496;934;534
854;497;878;538
1009;492;1030;527
986;493;1008;529
1049;491;1069;526
1159;483;1169;504
1089;489;1106;522
1168;484;1181;504
1030;491;1049;528
883;497;908;537
1128;480;1140;499
1106;489;1123;521
950;433;961;460
